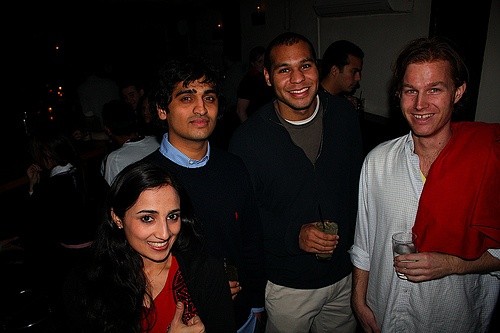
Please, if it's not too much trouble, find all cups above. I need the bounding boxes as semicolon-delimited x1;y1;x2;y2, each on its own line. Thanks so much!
313;221;339;260
392;232;418;279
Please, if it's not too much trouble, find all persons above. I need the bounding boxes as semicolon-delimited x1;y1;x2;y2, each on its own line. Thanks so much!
82;164;237;333
231;33;384;333
237;46;273;123
318;40;365;113
91;77;165;187
133;53;265;333
348;37;500;333
0;142;102;272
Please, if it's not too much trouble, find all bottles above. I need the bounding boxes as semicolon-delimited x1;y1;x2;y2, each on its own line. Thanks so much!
356;96;365;114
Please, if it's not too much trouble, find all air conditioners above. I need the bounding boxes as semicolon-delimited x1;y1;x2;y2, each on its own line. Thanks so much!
312;0;414;18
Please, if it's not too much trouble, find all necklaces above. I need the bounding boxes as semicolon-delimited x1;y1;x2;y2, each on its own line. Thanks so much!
146;260;169;288
